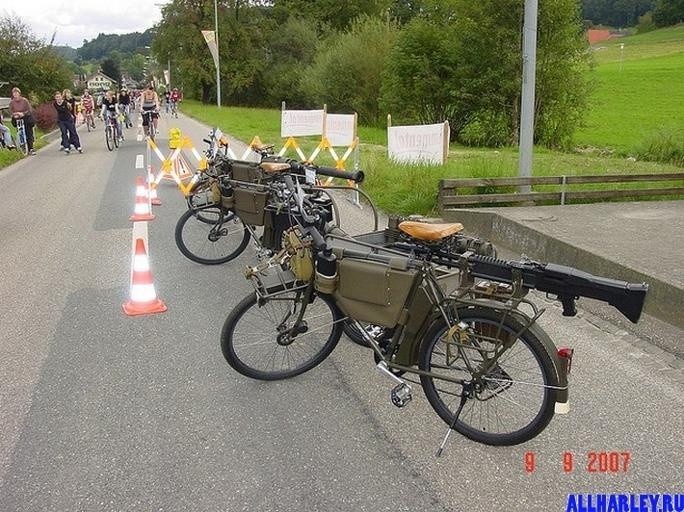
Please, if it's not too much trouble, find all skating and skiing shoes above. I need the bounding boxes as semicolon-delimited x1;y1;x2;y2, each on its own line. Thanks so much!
59;145;82;154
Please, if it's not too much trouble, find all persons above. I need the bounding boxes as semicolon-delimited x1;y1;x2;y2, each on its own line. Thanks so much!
53;83;183;154
0;114;15;148
9;87;36;155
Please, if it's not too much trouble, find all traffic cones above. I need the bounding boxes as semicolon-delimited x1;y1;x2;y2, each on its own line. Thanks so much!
122;165;167;316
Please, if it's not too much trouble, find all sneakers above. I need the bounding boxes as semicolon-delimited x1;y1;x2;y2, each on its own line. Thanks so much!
29;149;36;155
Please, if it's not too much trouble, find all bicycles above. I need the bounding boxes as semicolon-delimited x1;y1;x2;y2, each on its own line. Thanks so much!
174;128;574;457
11;114;37;157
81;99;183;151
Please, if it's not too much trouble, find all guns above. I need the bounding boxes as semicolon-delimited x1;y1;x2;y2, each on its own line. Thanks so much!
385;240;649;322
260;149;365;184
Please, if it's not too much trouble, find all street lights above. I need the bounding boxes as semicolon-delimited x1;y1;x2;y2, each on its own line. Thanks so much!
142;30;171;88
